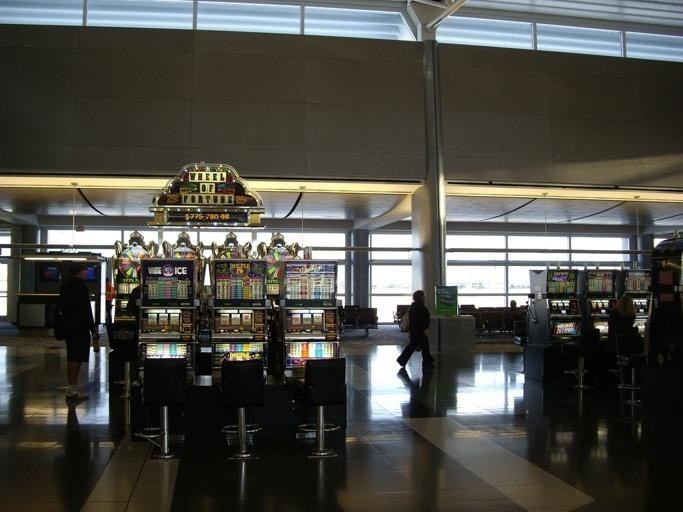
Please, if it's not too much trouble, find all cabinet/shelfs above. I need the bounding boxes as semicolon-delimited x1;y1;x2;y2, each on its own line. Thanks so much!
524;269;652;416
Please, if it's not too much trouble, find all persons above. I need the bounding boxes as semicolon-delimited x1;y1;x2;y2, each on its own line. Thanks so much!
126;270;142;316
62;264;99;402
106;276;116;322
397;289;437;370
505;301;522;321
608;295;637;350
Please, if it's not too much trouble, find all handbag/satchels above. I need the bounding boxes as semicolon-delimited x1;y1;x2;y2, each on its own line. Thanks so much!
399;314;409;331
52;310;66;340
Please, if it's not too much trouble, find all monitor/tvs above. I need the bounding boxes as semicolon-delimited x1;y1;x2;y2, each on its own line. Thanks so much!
41;264;61;281
80;265;98;281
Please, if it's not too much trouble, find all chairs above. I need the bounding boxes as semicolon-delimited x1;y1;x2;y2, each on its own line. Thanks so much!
338;305;378;337
135;359;347;460
394;305;530;334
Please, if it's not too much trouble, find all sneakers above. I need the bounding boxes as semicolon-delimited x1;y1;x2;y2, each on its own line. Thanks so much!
65;389;88;398
422;362;434;368
